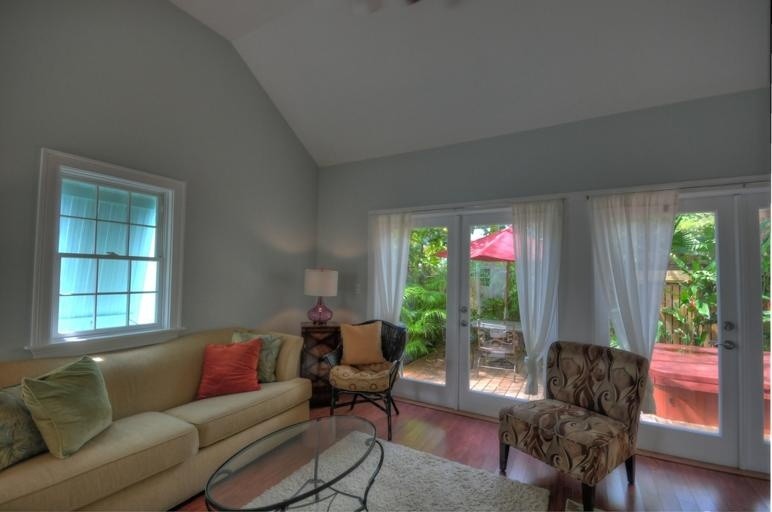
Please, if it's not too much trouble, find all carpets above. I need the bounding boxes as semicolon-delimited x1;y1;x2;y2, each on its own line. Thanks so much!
239;429;606;512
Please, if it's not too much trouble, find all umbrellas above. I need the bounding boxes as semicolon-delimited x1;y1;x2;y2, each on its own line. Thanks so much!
434;224;546;322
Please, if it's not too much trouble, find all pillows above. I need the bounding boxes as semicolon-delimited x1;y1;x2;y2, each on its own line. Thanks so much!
196;336;263;399
19;356;115;460
1;381;50;471
232;331;286;383
338;320;387;367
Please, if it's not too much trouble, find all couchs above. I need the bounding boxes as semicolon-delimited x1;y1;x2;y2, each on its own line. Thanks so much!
1;326;313;512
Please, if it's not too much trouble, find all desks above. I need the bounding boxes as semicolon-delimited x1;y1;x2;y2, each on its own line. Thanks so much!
471;317;522;338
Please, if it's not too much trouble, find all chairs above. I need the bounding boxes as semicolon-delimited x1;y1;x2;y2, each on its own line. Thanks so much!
329;318;408;442
474;319;523;384
498;341;650;511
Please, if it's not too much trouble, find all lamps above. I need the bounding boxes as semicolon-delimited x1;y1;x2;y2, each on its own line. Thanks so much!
303;266;340;325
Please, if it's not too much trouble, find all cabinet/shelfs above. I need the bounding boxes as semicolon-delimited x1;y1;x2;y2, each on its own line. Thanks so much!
300;323;341;409
